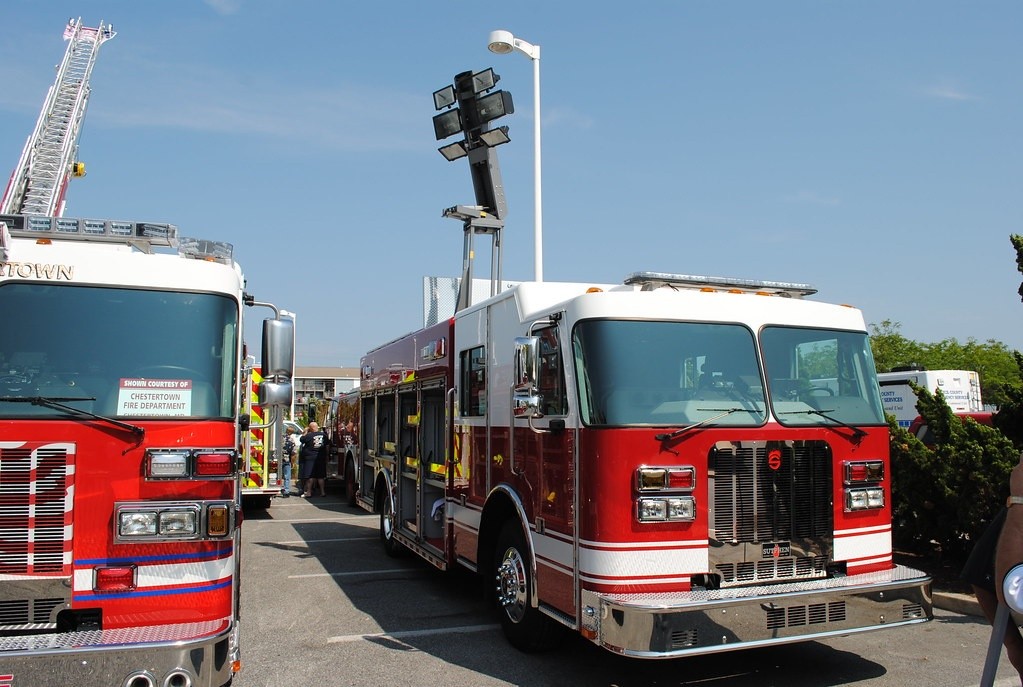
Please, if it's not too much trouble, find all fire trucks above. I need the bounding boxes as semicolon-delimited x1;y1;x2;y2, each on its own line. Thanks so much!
329;66;935;661
0;16;297;687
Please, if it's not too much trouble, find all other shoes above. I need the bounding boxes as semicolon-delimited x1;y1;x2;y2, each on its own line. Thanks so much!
283;494;289;498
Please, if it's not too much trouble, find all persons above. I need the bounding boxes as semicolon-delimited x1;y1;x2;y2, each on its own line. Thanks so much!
962;460;1023;687
282;421;331;498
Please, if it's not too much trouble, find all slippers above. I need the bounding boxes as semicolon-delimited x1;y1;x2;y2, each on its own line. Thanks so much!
299;494;312;498
320;494;327;497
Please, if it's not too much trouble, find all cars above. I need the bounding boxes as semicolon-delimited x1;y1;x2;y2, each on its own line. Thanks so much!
282;420;306;465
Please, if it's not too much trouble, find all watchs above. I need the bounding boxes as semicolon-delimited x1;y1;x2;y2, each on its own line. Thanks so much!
1006;496;1023;508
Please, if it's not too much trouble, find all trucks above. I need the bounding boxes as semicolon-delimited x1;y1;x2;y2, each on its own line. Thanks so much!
806;363;995;440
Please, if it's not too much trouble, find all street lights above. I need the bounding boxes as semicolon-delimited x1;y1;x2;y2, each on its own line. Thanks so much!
487;29;544;283
